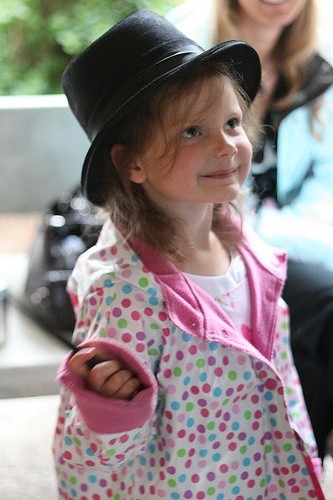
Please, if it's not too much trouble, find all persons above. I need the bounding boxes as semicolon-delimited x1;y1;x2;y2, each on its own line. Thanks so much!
209;0;333;470
51;9;327;500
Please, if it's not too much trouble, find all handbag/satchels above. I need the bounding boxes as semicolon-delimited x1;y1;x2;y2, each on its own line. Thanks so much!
22;189;105;332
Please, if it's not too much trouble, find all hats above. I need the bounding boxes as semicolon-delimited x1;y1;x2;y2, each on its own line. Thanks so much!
61;9;262;207
